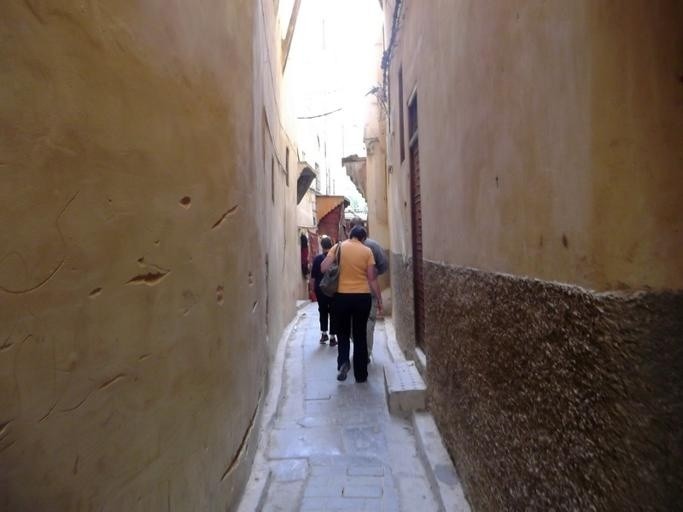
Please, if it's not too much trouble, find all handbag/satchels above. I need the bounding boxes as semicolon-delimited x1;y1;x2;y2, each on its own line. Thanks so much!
318;241;341;298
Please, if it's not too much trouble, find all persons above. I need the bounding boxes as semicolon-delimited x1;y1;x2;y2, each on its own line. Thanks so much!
309;216;389;383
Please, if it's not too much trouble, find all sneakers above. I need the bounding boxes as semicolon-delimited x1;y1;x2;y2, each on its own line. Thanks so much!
320;333;337;346
337;362;350;381
